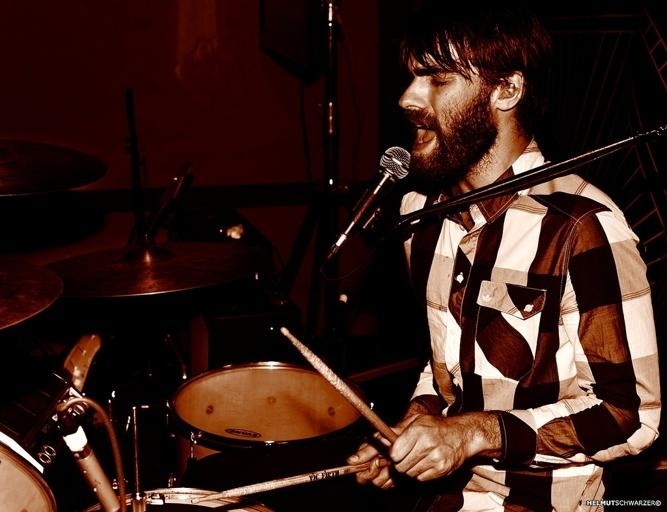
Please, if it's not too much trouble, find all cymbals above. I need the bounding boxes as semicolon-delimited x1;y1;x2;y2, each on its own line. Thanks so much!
45;244;252;300
0;141;106;196
0;254;63;329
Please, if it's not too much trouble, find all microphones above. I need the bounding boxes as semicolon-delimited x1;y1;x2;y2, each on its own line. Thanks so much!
328;146;412;262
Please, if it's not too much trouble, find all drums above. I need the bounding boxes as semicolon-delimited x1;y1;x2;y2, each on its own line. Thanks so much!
168;359;374;512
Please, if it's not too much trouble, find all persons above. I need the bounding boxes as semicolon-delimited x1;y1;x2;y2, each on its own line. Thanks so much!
346;13;662;512
